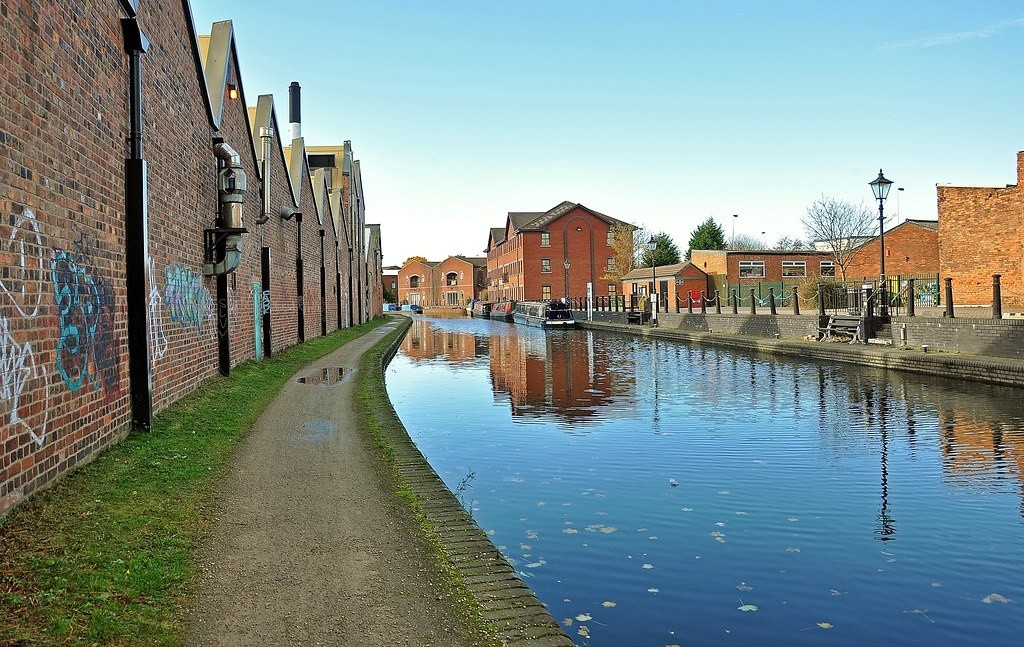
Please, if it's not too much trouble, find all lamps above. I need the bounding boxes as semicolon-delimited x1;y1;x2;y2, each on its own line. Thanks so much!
576;226;582;231
230;83;237;98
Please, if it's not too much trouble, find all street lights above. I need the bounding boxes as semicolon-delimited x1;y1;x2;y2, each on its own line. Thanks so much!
733;214;738;250
648;340;662;439
868;169;894;315
897;188;904;225
647;235;659;311
874;392;897;545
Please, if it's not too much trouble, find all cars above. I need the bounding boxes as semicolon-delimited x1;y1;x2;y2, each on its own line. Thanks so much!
917;284;930;297
931;284;940;293
921;293;937;305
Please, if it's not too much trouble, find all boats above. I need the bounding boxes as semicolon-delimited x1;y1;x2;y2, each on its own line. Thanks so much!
514;301;575;330
466;299;492;315
490;302;516;323
